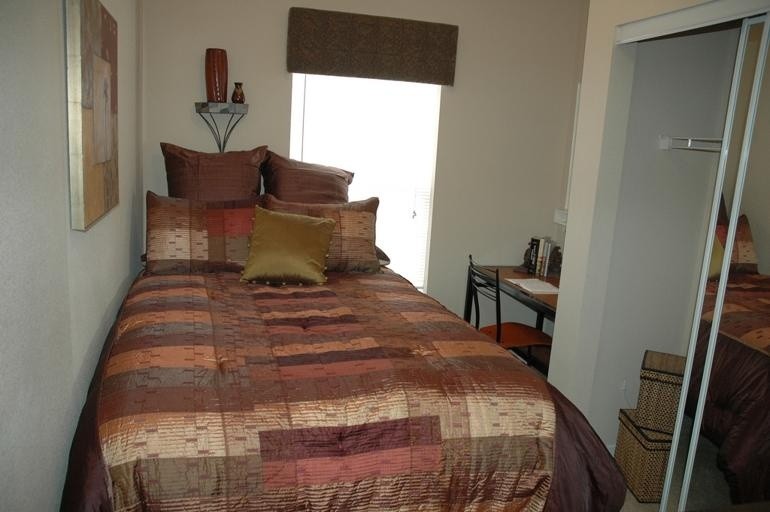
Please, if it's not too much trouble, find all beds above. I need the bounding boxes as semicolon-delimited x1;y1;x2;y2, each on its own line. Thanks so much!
58;265;565;512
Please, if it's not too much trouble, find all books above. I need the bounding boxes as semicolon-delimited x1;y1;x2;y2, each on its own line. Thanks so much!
503;278;558;295
527;236;561;277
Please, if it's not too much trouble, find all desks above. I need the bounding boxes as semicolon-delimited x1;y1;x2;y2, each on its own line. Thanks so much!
464;265;560;333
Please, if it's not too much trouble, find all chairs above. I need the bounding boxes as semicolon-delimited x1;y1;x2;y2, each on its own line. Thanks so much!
468;265;557;363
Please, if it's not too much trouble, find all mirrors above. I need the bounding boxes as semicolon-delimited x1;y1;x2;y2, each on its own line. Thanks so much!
659;12;770;512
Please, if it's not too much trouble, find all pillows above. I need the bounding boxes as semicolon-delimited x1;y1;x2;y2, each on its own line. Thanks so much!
141;140;390;285
